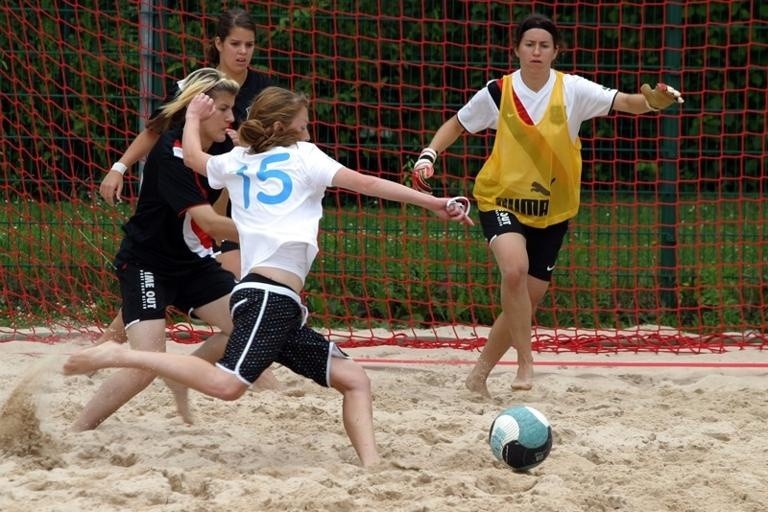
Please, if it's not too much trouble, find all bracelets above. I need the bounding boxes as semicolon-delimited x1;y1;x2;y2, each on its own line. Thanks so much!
110;162;127;175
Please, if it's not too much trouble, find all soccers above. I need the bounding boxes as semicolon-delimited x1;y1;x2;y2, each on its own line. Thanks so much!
490;405;553;470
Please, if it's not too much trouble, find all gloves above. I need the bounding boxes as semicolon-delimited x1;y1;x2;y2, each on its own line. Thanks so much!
413;147;438;195
640;83;684;112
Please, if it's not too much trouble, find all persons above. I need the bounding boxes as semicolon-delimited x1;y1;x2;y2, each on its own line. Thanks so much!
413;16;684;398
71;68;239;431
96;9;268;345
64;87;474;466
